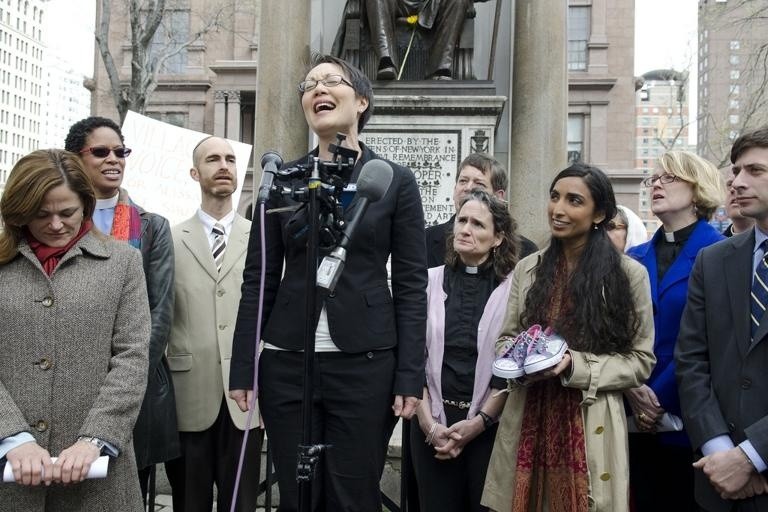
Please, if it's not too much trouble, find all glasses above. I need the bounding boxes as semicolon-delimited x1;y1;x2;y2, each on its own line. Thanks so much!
606;221;626;231
78;145;132;158
643;173;684;187
299;75;354;93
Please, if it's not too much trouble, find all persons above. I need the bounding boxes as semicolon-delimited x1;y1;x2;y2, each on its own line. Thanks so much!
721;169;754;239
676;130;764;510
401;154;540;511
230;54;426;512
366;0;477;82
63;116;183;509
607;204;648;254
410;190;520;510
622;149;731;509
166;135;271;512
1;149;152;510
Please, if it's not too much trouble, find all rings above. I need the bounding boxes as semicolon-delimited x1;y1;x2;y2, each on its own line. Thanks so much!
638;413;646;420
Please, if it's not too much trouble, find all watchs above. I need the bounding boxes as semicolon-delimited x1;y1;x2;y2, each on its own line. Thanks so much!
480;165;655;511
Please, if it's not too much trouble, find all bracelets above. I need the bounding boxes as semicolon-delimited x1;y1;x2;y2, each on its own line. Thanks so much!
424;422;440;446
77;436;105;452
476;409;494;430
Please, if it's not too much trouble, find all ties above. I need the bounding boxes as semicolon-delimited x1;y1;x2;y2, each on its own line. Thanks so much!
212;223;227;275
750;240;767;343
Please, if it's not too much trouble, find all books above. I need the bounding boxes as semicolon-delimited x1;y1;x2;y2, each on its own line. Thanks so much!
3;453;113;486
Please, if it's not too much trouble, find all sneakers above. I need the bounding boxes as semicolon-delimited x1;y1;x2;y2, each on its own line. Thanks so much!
524;326;568;375
492;324;543;379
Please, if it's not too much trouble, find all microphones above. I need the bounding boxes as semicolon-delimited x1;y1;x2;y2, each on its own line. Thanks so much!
331;158;395;262
257;150;284;206
